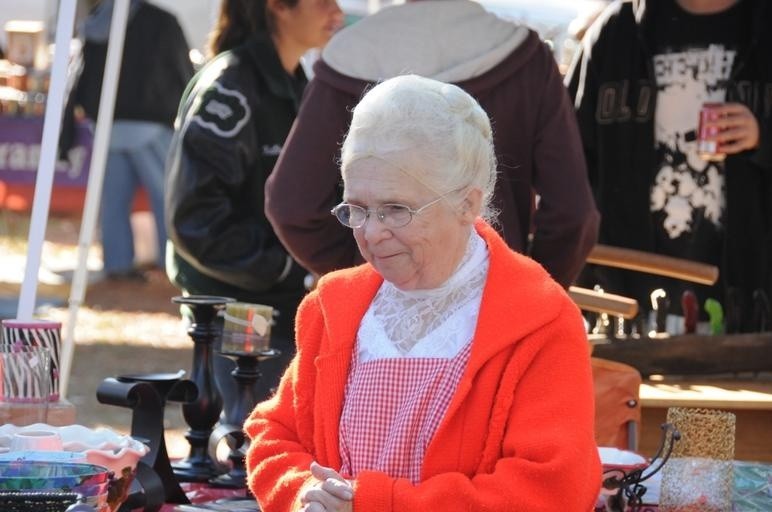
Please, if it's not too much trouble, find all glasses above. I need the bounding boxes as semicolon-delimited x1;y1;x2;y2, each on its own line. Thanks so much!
331;187;466;229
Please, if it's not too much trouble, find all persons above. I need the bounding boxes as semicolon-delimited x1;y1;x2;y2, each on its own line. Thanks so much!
48;0;200;290
550;0;772;358
258;0;605;310
236;71;606;511
158;0;349;430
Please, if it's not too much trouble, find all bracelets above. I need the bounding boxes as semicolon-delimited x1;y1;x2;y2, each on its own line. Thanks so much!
304;274;314;290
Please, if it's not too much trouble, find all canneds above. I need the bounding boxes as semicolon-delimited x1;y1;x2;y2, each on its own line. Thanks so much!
698;101;728;162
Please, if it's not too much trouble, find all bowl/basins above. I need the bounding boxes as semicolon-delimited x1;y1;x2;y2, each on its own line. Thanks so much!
594;446;648;495
0;460;112;511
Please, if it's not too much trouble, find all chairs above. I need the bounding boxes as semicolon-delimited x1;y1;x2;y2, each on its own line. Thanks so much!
591;355;642;512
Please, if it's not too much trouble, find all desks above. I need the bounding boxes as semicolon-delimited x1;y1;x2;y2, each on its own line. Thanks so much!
628;378;772;468
0;113;154;216
134;457;772;512
